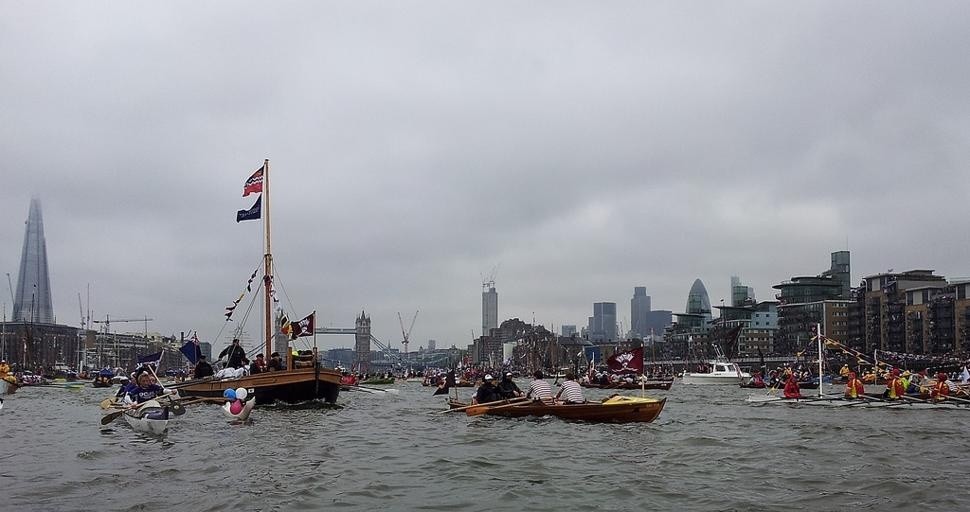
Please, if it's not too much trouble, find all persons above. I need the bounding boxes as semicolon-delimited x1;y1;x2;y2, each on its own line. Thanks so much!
752;364;949;400
0;359;16;383
525;370;554;405
193;339;282;379
477;372;524;402
556;373;584;403
116;371;177;409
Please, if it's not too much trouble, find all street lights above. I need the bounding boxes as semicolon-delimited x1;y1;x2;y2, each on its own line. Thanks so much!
718;298;727;335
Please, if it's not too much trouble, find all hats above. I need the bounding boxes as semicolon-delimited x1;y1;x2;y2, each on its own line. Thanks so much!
234;338;239;343
256;353;263;357
506;372;512;377
847;372;854;378
845;364;848;367
1;359;5;363
937;373;946;381
889;368;926;379
786;371;792;375
271;352;280;357
482;373;493;381
199;355;206;359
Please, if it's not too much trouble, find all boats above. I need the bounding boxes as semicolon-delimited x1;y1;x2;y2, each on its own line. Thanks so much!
447;384;667;424
335;323;675;391
747;321;970;412
682;341;901;392
122;392;173;437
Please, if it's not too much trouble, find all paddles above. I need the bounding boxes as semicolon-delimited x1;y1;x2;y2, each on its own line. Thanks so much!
746;394;820;403
438;396;532;415
100;365;185;425
19;379;93;392
342;384;400;397
858;394;932;409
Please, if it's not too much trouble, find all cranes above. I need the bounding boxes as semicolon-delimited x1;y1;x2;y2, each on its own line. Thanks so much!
396;307;421;352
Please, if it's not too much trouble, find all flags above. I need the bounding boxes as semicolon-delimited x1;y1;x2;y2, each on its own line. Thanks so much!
237;193;262;223
242;164;262;197
290;313;314;338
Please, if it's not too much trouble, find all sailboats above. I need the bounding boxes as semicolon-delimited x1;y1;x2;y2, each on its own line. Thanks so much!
1;159;345;405
